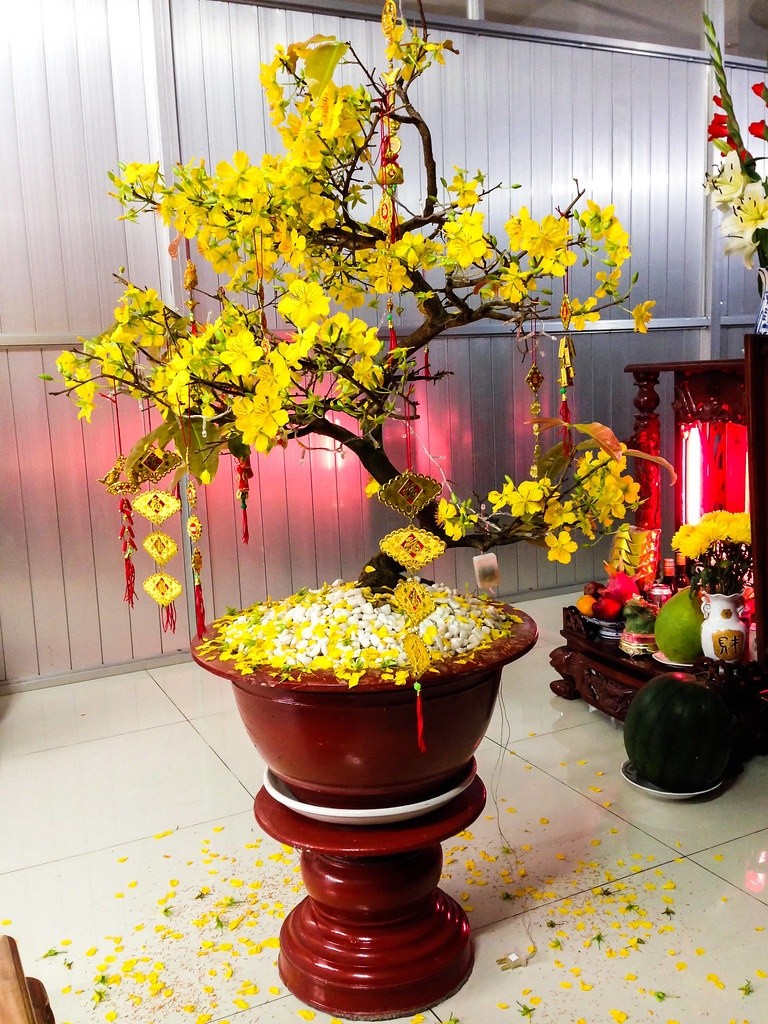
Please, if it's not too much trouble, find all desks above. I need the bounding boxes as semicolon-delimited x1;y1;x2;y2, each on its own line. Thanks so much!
622;358;748;586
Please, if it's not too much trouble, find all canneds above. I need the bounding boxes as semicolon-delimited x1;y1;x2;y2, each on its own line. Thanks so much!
648;583;672;609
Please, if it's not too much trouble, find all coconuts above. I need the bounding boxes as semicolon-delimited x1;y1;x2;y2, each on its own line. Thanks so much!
655;586;706;664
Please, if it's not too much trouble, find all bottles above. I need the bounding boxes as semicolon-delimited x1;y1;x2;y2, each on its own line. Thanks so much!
662;558;678;599
675;553;691;592
748;623;757;663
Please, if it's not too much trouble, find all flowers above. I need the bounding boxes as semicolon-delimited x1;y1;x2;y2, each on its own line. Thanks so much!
670;510;753;601
701;10;768;299
37;0;678;672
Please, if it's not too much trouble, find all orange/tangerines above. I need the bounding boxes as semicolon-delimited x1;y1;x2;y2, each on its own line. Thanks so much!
576;595;598;617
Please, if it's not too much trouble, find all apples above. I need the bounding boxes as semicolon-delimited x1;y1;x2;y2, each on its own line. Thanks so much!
592;597;625;622
584;582;605;599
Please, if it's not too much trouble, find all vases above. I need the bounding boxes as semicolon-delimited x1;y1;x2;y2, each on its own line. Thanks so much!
700;590;748;673
189;600;540;1023
753;266;768;335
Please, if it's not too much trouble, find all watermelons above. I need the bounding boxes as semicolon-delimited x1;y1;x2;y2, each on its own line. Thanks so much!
623;672;734;793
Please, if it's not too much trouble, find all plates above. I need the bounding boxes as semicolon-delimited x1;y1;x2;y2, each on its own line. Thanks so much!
651;651;708;670
619;760;723;800
